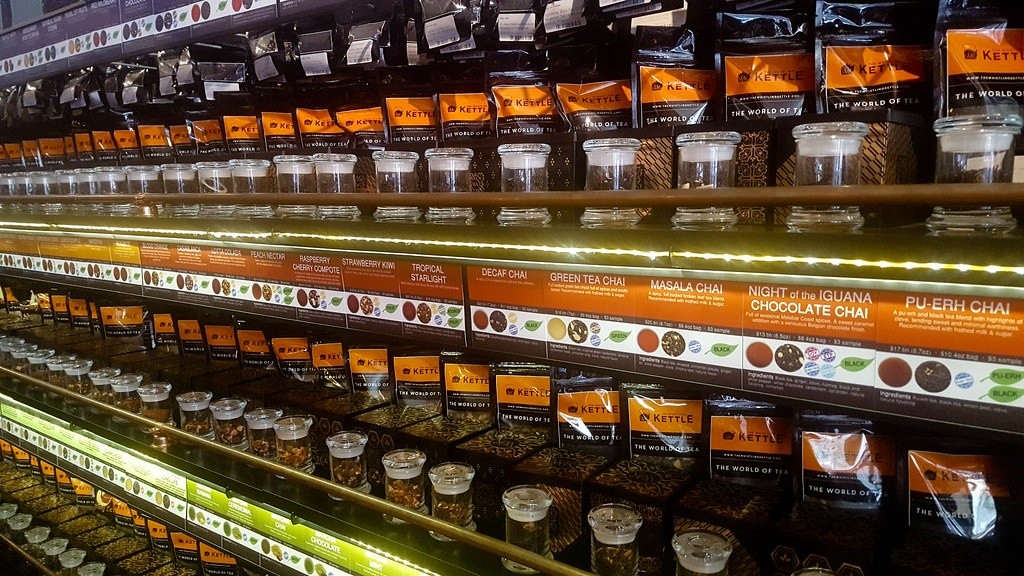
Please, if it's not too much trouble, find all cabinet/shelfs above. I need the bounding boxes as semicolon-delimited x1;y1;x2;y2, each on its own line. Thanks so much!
0;0;1024;576
